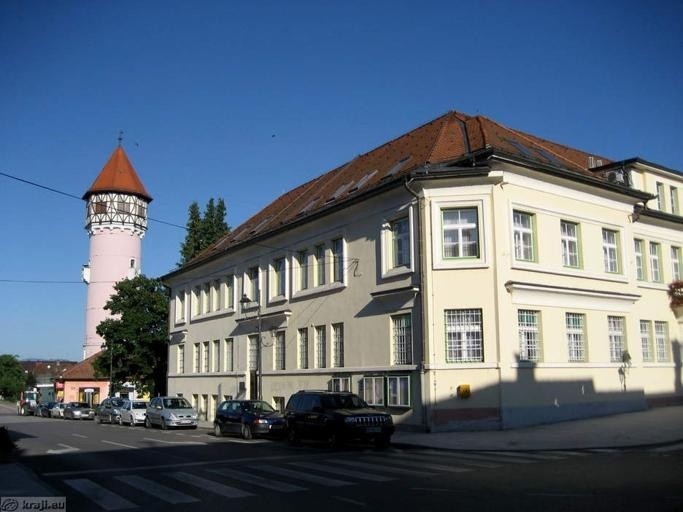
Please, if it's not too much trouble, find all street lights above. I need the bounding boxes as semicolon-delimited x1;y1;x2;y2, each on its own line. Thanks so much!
239;289;262;400
81;339;112;396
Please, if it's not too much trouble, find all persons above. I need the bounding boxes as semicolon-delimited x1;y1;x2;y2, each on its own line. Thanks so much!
35;388;42;416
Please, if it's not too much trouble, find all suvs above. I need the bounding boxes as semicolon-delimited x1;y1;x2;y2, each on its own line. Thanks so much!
94;397;129;423
284;389;395;449
214;399;285;440
144;396;199;431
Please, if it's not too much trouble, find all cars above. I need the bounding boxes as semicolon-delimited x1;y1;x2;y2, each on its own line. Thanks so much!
120;400;149;427
34;400;96;422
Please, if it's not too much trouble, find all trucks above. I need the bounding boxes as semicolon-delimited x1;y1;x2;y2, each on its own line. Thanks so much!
16;390;42;417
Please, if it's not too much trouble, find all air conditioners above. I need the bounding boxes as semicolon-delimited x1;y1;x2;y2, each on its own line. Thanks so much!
606;171;625;184
